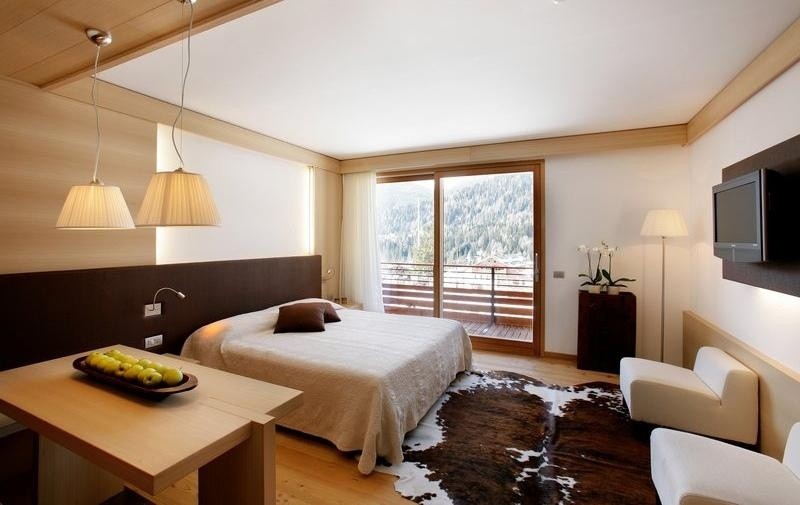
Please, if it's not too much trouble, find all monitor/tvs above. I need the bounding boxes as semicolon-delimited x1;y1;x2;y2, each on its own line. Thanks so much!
712;168;763;264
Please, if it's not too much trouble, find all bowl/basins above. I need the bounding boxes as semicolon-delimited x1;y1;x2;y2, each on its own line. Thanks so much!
73;354;200;402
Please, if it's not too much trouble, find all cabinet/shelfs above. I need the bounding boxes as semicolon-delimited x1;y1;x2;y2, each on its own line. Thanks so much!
577;289;637;375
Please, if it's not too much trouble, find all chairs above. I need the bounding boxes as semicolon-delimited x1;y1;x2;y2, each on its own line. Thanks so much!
619;346;760;447
649;422;800;505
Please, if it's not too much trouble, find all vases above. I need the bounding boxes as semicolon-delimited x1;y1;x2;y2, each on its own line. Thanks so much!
588;285;601;294
607;286;619;295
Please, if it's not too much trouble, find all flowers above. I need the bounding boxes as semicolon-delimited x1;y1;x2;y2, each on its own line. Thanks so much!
576;244;604;286
601;240;636;288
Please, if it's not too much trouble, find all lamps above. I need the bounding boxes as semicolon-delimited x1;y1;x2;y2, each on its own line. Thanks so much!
53;26;135;231
133;0;223;228
639;210;689;364
321;267;335;281
149;287;186;310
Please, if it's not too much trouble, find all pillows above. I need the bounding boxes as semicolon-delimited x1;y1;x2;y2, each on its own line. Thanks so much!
273;303;327;333
324;302;342;323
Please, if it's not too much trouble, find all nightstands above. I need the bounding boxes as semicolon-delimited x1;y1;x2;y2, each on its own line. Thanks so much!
329;298;364;310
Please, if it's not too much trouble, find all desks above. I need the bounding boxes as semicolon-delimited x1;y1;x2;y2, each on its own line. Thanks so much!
0;343;305;505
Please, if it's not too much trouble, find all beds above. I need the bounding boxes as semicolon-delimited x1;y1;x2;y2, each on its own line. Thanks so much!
180;297;473;475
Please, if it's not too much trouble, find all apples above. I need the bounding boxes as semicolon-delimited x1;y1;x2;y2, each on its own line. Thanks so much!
85;350;184;384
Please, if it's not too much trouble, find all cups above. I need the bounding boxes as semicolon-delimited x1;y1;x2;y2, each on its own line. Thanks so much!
342;298;347;305
335;298;339;304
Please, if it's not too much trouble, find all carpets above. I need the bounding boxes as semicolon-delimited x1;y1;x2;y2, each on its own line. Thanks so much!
373;366;660;505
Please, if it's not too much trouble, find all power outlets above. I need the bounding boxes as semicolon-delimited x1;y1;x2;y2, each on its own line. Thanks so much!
145;335;163;348
145;303;161;317
